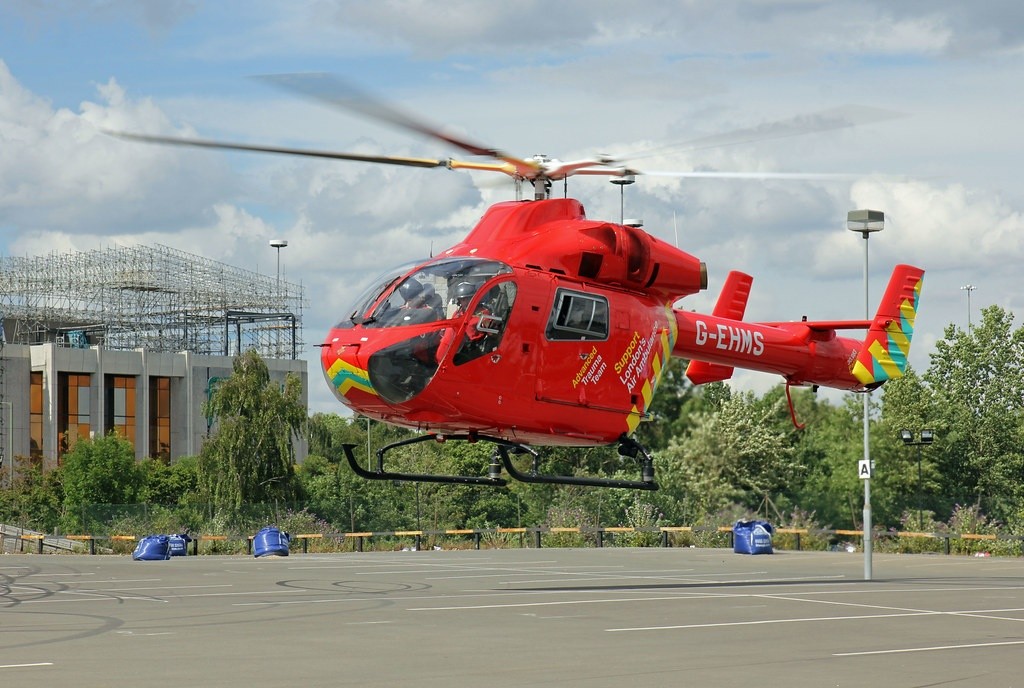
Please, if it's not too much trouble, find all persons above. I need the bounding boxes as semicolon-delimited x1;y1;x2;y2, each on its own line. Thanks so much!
433;282;491;365
398;279;442;363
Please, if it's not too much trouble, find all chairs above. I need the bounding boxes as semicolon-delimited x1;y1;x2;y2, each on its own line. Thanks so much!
475;276;501;305
423;283;444;319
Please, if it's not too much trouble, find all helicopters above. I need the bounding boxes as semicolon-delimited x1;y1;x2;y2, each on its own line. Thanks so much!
102;71;927;494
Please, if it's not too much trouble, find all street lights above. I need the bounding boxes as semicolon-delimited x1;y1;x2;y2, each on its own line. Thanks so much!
848;209;885;583
960;283;978;337
900;428;935;533
269;239;288;360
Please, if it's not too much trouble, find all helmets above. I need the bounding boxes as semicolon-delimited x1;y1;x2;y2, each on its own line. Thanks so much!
399;278;423;300
455;282;475;298
